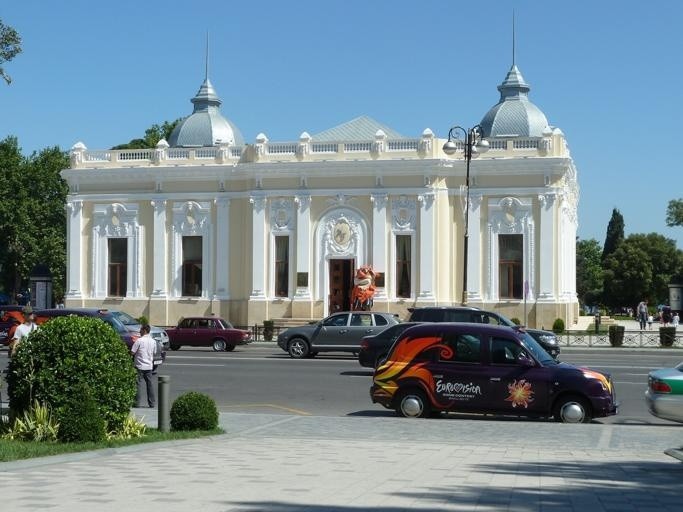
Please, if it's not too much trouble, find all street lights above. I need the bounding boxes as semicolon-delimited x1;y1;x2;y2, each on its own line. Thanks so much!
14;260;18;302
442;125;490;306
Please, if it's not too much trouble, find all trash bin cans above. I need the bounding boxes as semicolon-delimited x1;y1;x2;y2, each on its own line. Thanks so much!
609;325;625;347
659;327;675;346
263;320;274;340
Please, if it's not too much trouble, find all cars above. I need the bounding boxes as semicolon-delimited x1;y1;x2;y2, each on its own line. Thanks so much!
0;305;251;375
645;361;683;423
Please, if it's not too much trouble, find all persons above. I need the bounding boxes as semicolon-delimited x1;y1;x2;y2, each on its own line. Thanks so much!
646;312;653;329
636;299;647;330
657;303;680;327
127;324;156;408
10;306;38;357
